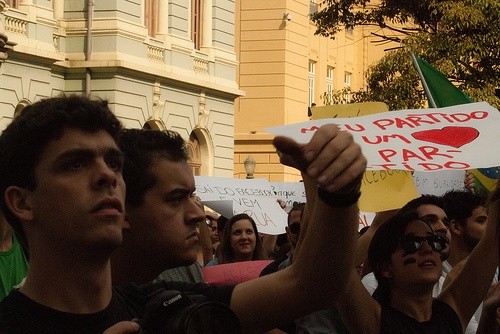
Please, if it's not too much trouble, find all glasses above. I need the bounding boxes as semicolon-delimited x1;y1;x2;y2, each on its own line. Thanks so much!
394;231;449;253
286;222;300;234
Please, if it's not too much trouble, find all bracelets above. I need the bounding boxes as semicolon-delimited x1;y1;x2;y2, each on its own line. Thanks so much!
318;179;361;207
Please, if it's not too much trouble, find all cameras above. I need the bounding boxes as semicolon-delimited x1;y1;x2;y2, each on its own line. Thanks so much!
138;288;242;334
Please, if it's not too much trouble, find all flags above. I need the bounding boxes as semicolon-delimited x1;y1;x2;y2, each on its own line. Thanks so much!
413;56;500;193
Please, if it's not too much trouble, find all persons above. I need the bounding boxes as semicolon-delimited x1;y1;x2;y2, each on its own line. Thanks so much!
0;145;500;334
109;129;285;334
0;93;367;334
